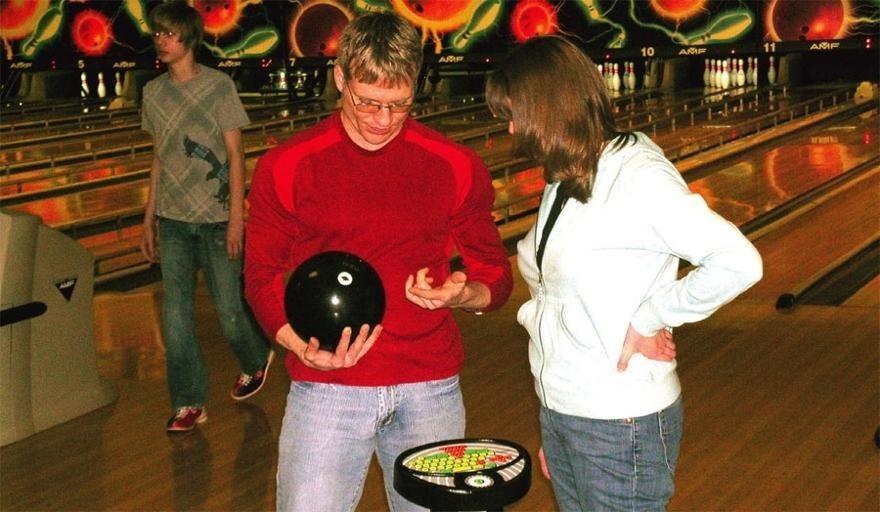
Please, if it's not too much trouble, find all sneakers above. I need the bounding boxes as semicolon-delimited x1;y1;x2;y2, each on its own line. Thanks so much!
230;350;274;401
165;404;207;432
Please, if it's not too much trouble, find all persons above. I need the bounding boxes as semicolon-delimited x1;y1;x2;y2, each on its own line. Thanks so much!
484;33;763;511
244;9;515;512
137;3;275;432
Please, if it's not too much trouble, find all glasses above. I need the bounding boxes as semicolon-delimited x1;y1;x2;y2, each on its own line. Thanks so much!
345;80;418;113
